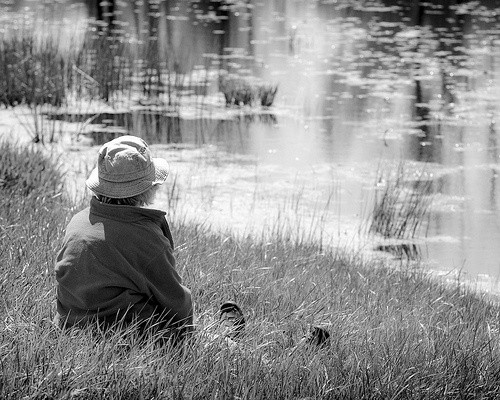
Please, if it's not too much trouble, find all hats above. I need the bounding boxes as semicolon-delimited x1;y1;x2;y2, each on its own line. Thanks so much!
86;134;169;199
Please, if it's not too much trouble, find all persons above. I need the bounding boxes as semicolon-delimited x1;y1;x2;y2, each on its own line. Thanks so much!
54;135;198;351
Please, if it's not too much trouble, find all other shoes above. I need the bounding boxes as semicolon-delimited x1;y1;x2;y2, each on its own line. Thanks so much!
219;301;244;339
292;326;330;355
